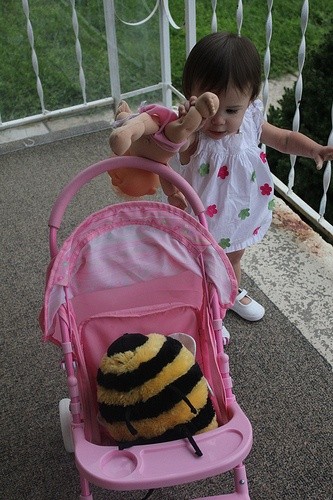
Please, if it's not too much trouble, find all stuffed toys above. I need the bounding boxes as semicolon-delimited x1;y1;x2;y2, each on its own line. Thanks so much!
95;332;220;458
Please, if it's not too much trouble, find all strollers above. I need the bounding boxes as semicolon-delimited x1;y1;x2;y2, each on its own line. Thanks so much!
39;154;253;500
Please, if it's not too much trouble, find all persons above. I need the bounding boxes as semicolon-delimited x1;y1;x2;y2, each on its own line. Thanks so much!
178;31;332;348
106;92;219;211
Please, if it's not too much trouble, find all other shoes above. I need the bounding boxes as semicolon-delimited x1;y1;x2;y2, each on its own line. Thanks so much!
221;321;231;346
227;288;266;322
